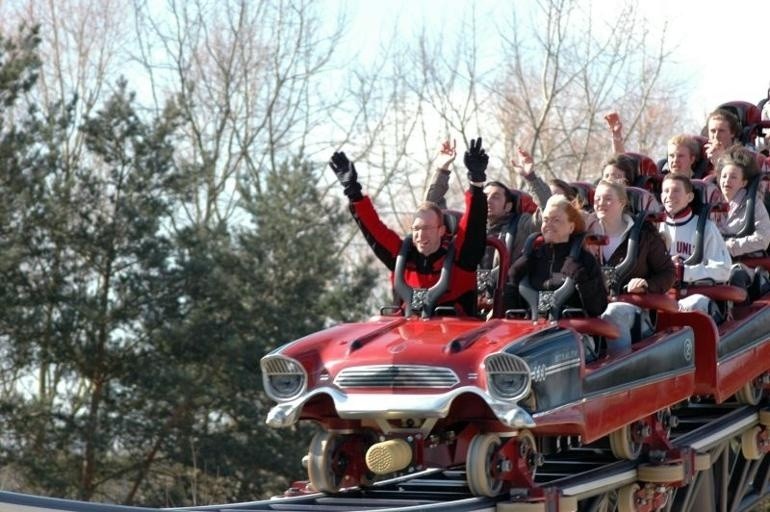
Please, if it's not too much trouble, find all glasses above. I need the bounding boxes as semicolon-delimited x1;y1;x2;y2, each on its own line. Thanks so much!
409;224;439;232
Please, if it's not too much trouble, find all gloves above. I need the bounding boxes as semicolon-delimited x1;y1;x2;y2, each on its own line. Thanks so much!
463;136;489;182
329;151;361;195
